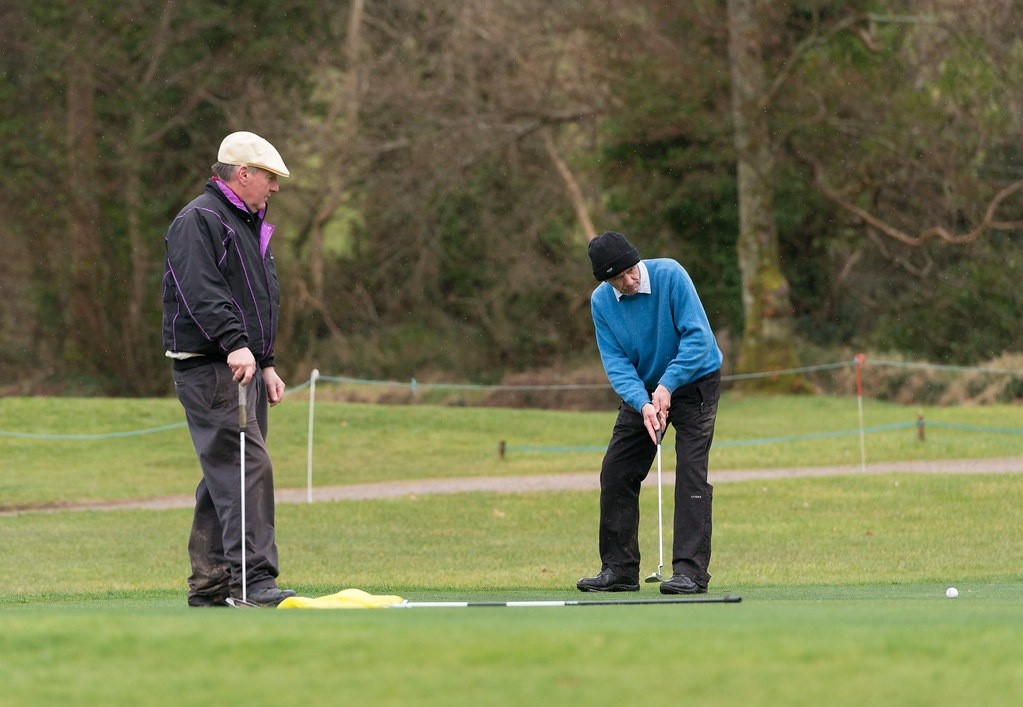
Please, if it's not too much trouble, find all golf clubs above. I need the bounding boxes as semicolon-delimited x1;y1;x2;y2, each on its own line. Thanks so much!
644;411;664;583
224;371;263;609
395;595;742;609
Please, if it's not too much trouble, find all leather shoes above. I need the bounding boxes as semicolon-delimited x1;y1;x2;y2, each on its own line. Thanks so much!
188;592;230;607
230;577;295;607
577;568;640;593
660;574;707;594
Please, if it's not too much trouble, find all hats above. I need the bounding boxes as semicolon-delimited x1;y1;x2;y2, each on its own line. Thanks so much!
588;231;641;281
217;132;289;178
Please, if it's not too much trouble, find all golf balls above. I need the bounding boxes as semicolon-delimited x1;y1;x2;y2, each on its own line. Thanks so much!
945;587;959;599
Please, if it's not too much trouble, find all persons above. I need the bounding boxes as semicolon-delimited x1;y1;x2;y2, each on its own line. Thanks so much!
576;232;724;595
163;131;295;607
